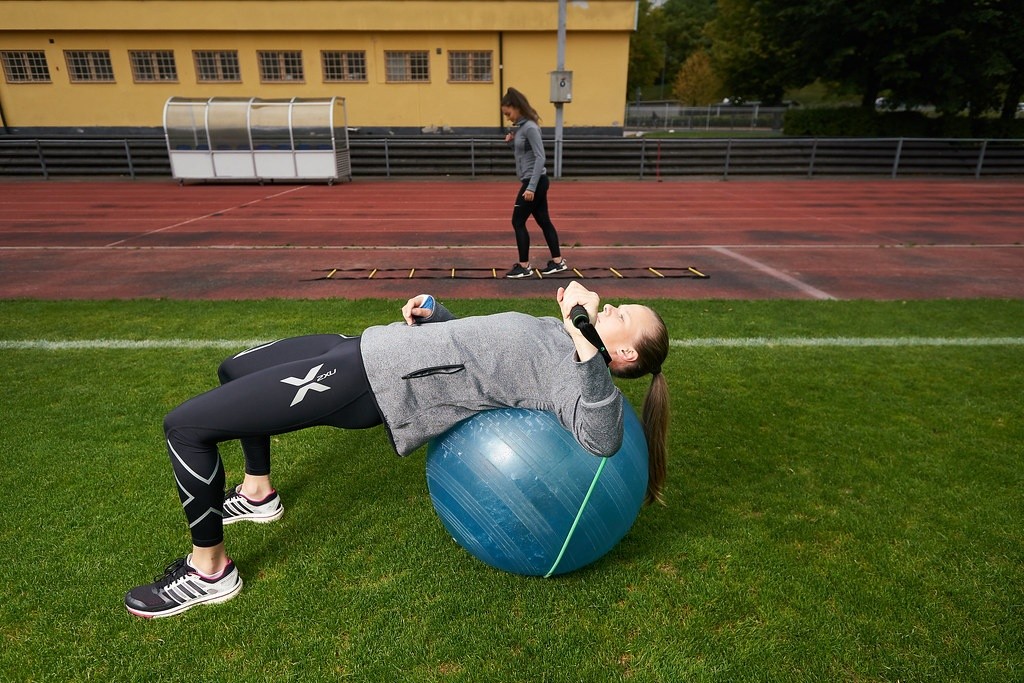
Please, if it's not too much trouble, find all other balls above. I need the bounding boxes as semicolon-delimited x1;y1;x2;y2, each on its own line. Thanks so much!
423;386;651;580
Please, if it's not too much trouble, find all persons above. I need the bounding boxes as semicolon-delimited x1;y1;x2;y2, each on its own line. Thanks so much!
501;87;568;278
123;280;669;619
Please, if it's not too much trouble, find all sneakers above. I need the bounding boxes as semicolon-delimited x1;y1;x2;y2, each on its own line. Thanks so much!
221;482;285;525
541;258;567;274
123;552;243;620
506;263;533;279
